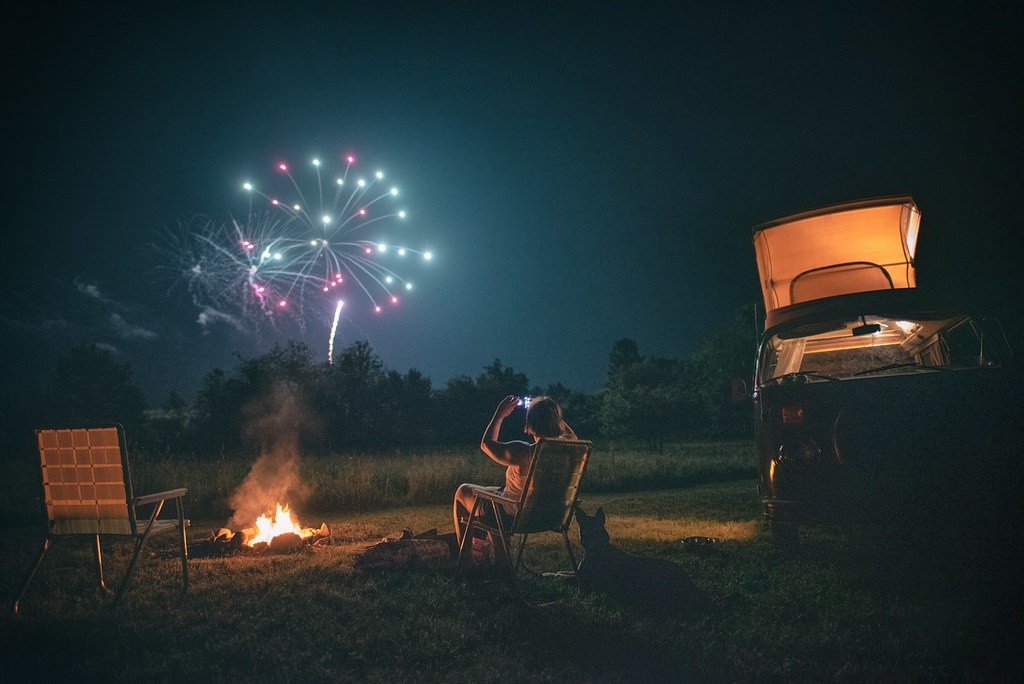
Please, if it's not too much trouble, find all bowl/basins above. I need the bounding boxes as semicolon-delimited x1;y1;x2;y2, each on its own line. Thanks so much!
682;536;717;549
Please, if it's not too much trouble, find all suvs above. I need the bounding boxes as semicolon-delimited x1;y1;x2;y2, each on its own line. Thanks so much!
749;192;1024;559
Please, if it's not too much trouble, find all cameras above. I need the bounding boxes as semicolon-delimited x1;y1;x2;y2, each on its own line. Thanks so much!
516;397;532;408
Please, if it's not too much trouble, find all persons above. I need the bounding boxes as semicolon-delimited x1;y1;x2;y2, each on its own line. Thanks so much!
453;395;578;576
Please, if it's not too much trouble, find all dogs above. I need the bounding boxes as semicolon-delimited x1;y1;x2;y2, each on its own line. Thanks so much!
543;507;713;613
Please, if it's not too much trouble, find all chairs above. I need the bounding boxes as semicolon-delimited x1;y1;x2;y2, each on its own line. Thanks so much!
10;421;190;625
451;437;593;608
